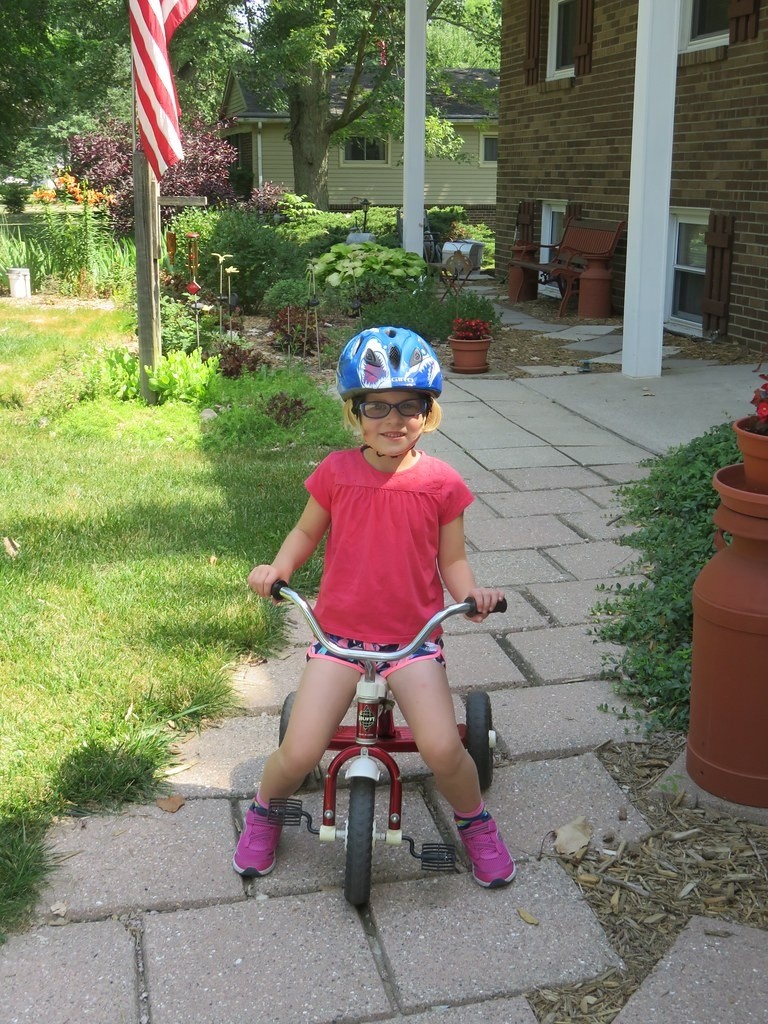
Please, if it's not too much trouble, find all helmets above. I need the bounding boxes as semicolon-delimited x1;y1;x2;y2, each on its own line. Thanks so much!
336;326;442;402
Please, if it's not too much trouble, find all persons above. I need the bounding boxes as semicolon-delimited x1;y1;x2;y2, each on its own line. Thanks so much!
231;324;519;890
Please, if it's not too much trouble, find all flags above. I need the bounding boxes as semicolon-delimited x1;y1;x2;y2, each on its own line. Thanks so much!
128;0;201;184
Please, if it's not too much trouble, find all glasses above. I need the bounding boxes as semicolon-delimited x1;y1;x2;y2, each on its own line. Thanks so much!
359;397;429;418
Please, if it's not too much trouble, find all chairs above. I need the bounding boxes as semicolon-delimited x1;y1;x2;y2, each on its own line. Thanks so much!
396;209;441;260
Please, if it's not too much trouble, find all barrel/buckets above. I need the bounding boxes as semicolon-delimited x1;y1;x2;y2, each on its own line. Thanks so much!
9;268;30;298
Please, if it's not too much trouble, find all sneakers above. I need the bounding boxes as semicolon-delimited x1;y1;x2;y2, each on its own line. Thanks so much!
453;812;517;889
232;797;283;878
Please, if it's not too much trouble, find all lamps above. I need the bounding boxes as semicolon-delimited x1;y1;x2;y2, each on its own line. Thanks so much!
361;200;371;212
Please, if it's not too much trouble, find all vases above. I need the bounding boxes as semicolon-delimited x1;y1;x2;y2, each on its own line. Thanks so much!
732;416;768;492
448;336;493;372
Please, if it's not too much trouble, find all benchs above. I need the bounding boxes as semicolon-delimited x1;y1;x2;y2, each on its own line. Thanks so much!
510;215;626;317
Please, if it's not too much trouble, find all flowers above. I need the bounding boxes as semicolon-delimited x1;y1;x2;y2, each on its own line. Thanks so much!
750;374;768;432
447;318;491;340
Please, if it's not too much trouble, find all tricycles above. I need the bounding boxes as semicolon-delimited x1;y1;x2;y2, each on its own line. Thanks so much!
260;578;508;909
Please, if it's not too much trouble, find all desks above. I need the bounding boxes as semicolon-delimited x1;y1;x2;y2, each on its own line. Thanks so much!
427;262;479;301
